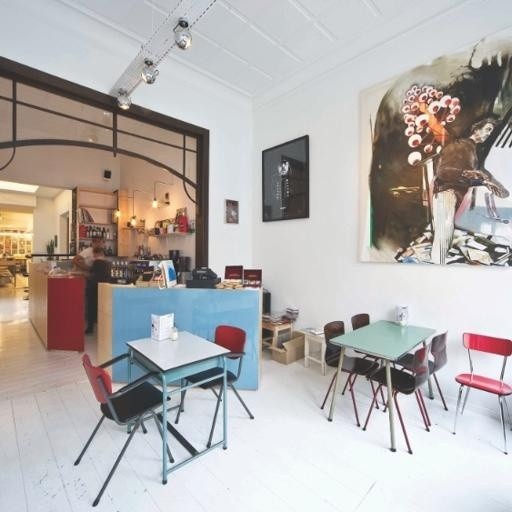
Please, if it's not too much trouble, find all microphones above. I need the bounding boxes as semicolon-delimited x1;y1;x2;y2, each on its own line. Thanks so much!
262;322;293;356
303;331;328;375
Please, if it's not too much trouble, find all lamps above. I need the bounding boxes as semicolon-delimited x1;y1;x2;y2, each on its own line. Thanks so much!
73;354;174;507
321;321;380;425
175;324;254;449
453;330;511;454
382;330;449;413
363;342;431;455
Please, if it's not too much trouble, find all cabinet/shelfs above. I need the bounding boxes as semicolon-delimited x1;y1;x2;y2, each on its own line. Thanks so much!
178;207;188;232
108;246;113;256
92;226;96;238
86;226;89;238
104;245;107;257
102;228;106;238
106;228;109;240
110;261;128;281
89;226;92;238
97;227;102;238
79;241;92;252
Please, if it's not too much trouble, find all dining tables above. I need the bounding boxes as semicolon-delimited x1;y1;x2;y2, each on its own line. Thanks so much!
46;239;55;261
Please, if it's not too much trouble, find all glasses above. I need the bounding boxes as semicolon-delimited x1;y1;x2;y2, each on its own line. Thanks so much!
151;180;170;210
129;189;139;226
113;189;122;217
118;87;132;111
171;16;192;53
141;58;158;86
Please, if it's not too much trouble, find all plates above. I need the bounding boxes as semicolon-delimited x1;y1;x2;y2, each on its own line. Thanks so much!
73;186;130;258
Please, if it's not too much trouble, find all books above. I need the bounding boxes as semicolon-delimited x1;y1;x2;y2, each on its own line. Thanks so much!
267;330;305;366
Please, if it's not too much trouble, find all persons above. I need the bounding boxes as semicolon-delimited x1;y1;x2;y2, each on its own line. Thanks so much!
89;249;120;299
268;152;302;219
430;118;504;267
74;235;107;332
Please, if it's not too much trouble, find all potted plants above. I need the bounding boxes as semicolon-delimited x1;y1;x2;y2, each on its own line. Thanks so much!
261;133;310;223
225;198;239;224
356;58;512;270
